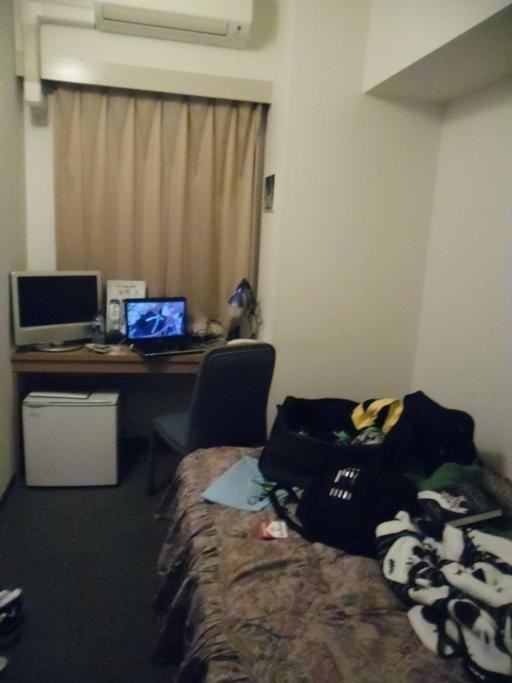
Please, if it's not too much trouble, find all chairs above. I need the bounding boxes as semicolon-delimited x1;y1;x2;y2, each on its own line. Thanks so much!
142;337;276;492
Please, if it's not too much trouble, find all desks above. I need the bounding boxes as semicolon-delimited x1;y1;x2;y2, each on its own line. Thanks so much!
9;346;204;491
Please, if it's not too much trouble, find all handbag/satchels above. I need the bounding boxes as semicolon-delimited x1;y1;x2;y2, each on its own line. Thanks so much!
257;395;384;508
384;390;478;479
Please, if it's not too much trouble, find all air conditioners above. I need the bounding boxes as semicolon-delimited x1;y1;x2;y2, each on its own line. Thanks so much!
91;1;257;52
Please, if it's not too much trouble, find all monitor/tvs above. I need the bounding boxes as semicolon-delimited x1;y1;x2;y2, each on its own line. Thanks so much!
124;298;206;358
9;271;102;354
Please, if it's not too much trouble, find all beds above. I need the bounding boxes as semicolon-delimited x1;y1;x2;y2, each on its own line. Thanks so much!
151;443;511;682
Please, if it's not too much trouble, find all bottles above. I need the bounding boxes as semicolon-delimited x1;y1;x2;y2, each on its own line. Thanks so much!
92;311;103;345
109;299;121;333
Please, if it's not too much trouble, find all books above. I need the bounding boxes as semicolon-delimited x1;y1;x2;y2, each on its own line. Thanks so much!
419;483;503;529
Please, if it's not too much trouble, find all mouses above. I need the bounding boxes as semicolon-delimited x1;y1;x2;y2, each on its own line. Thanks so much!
95;345;112;353
